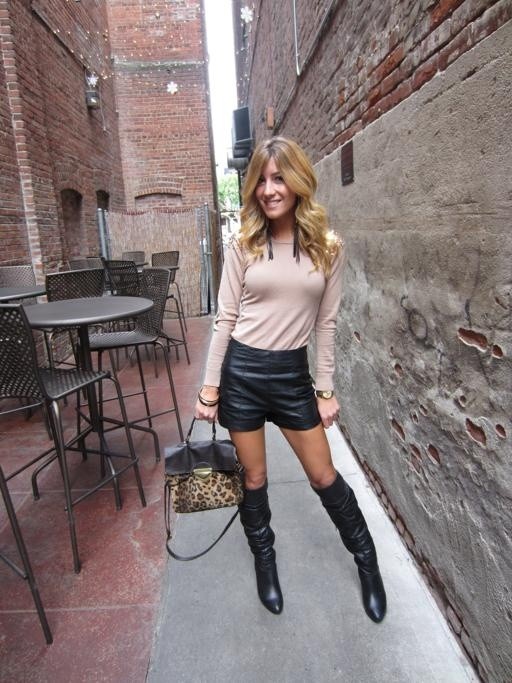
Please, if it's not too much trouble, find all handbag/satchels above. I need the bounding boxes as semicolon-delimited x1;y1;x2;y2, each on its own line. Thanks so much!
198;388;221;406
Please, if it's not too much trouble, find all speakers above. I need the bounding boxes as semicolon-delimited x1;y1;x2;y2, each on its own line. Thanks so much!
235;477;282;614
312;468;386;623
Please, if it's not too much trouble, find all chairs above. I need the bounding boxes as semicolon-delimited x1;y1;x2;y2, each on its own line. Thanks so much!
0;250;191;378
80;267;185;442
2;303;147;575
2;472;54;643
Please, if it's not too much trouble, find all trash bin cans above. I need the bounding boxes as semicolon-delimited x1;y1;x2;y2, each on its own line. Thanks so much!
316;390;334;399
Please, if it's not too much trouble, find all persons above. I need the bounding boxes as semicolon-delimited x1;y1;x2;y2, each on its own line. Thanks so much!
193;136;387;623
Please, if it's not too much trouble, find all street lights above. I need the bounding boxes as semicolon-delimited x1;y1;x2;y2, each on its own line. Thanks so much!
165;439;244;512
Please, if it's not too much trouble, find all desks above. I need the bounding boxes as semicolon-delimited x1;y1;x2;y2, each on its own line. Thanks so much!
20;295;161;510
